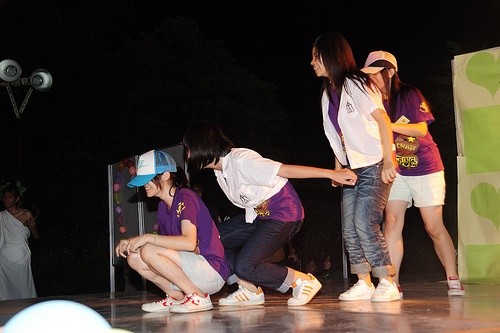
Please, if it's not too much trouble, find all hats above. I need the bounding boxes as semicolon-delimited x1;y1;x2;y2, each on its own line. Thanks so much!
127;149;177;187
360;51;398;74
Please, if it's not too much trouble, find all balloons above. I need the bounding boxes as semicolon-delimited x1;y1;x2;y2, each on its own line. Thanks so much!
2;300;112;333
114;158;137;238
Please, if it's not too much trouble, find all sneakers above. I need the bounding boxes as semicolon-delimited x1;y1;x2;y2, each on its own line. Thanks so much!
339;280;376;301
287;273;322;305
143;313;173;328
339;301;376;313
165;311;212;325
372;302;401;314
288;306;324;329
218;285;265;306
219;307;265;328
370;279;401;302
447;276;465;295
169;293;213;313
397;285;403;297
142;294;187;313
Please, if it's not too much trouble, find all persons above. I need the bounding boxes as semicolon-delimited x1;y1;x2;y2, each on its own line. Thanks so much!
311;33;401;302
0;181;343;300
116;150;230;313
360;50;465;297
184;121;358;307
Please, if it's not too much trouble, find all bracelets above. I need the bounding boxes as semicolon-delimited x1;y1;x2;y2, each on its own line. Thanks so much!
152;234;158;247
